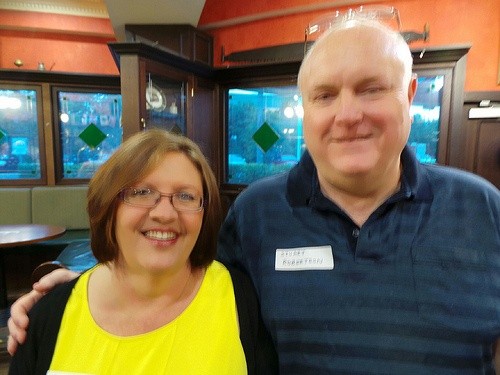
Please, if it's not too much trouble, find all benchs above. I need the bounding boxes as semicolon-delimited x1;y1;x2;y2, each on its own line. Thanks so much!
0;186;100;274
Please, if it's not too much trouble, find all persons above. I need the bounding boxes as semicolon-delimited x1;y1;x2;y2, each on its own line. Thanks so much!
7;18;499;372
8;130;279;372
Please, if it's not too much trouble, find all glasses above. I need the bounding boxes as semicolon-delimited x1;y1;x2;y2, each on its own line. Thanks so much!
118;187;205;212
303;4;403;58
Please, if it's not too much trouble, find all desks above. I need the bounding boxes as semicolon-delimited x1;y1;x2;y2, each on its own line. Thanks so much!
0;225;67;327
